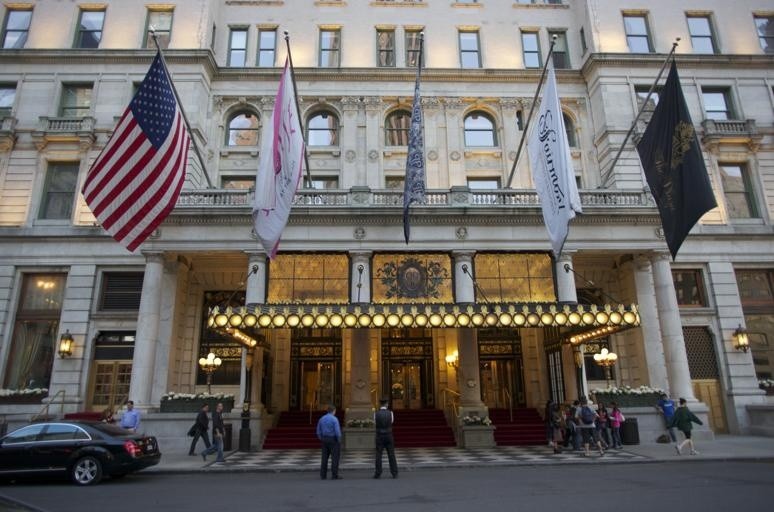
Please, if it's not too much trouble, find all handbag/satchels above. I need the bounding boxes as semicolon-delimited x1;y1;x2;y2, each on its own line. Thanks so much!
187;422;197;437
654;433;672;444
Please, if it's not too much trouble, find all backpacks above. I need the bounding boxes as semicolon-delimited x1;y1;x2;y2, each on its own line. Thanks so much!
579;404;594;425
617;410;626;422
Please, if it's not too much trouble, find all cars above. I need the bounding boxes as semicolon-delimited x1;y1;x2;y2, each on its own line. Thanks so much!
0;417;161;486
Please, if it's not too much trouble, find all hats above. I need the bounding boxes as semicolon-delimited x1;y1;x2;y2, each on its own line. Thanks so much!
379;396;389;404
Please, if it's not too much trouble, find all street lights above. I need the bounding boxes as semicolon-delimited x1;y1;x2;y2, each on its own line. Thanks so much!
594;346;620;387
198;351;224;394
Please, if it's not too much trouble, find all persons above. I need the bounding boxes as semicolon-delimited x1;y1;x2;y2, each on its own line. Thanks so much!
118;400;141;433
666;397;703;455
656;393;677;445
200;402;226;464
374;397;399;479
189;405;211;456
544;395;625;458
316;405;344;480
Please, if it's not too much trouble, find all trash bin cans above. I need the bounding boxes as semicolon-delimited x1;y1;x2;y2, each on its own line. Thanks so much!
620;417;640;445
213;424;232;451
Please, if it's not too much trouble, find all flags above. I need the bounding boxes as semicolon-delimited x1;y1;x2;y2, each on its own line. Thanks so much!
526;55;583;261
637;59;718;264
80;50;192;253
402;60;428;245
251;56;307;260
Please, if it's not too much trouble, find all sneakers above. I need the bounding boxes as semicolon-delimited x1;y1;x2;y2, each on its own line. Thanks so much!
674;443;682;455
688;450;700;455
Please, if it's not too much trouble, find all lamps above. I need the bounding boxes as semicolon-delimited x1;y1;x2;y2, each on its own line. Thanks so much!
733;322;751;354
57;328;74;361
